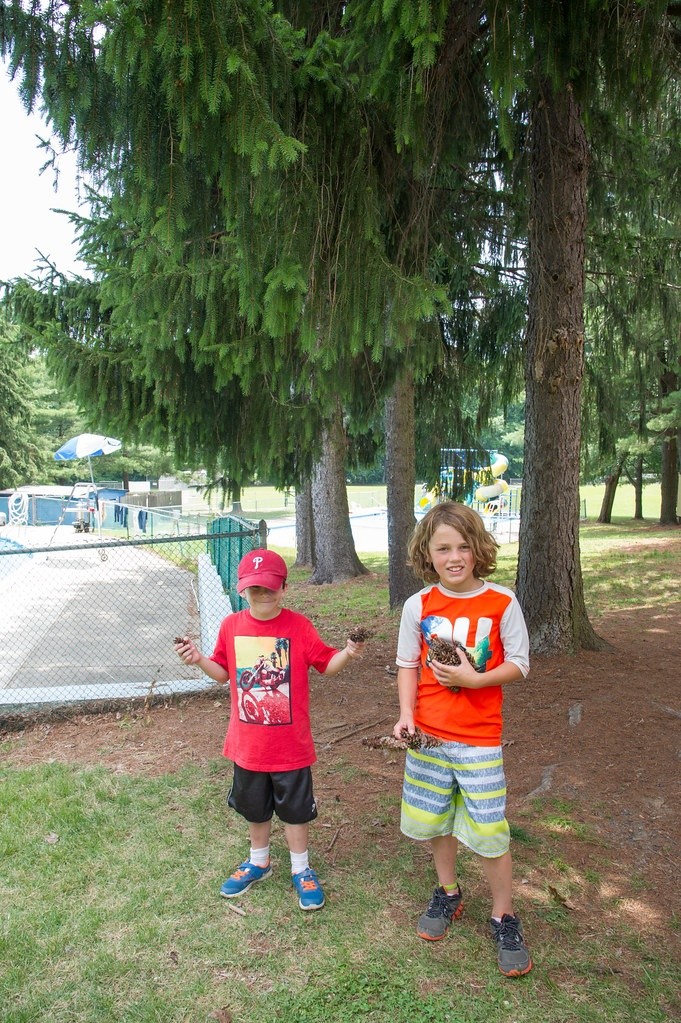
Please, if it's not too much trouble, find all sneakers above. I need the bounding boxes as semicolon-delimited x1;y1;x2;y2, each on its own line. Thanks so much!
489;914;532;976
418;884;464;941
220;860;274;898
290;868;325;909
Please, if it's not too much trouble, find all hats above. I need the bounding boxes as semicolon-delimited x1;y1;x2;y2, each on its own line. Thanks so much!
236;550;287;592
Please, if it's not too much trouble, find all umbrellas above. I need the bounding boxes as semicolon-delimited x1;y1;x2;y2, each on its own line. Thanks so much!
54;433;123;485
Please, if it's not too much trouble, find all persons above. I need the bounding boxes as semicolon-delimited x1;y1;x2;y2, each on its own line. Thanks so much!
172;548;367;912
391;502;533;978
